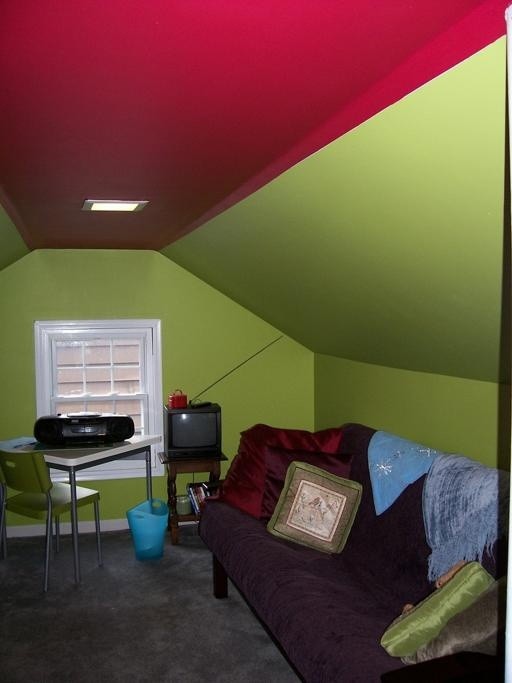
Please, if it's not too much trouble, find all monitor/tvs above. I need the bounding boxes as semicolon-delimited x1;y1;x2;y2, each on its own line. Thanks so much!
162;402;222;459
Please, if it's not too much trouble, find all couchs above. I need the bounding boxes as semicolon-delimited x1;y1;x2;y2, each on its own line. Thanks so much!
201;421;510;683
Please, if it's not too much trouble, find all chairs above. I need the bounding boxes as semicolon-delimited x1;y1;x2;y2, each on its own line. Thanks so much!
0;448;100;597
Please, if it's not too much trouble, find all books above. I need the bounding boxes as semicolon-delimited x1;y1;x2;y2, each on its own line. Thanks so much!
186;479;216;514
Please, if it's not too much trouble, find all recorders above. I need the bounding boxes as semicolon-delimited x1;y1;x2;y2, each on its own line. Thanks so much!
32;410;135;445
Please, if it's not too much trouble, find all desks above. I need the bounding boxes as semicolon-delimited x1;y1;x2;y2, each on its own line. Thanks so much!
0;435;162;591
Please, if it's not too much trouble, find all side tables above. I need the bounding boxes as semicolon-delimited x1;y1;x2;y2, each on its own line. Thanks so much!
157;452;228;546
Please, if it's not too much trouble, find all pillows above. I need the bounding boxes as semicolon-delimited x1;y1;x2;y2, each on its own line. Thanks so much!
261;442;353;528
381;561;494;658
267;461;363;554
401;578;507;665
216;424;356;522
